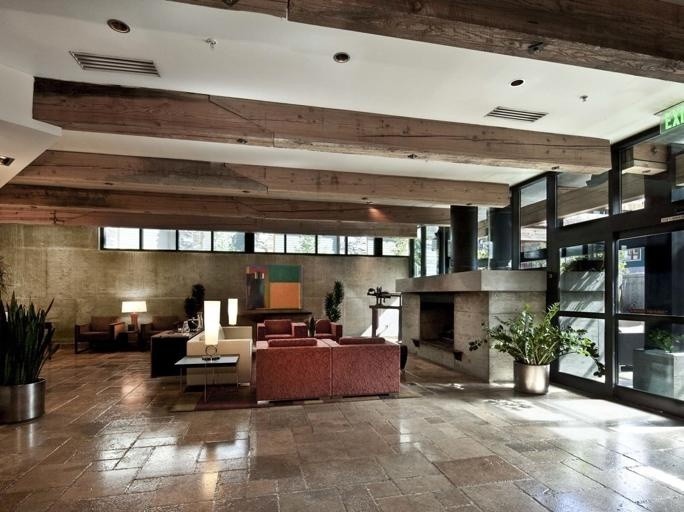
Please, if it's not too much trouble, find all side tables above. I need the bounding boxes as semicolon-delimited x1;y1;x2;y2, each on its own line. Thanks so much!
119;330;138;351
175;354;240;403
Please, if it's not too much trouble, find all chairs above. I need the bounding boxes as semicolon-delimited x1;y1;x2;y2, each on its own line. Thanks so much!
141;317;179;351
75;315;125;354
255;319;401;402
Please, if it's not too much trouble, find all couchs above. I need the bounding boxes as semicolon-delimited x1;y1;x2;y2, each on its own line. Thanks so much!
187;327;252;387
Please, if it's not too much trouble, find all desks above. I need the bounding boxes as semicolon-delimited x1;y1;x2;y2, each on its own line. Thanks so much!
150;329;202;376
238;309;312;323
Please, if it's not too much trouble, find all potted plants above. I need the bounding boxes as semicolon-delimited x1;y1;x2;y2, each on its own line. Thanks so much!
185;285;203;332
468;304;606;395
561;252;625;292
631;329;684;401
0;290;60;425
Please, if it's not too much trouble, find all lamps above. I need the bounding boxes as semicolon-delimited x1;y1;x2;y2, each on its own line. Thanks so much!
203;301;219;360
121;301;146;332
227;298;238;326
